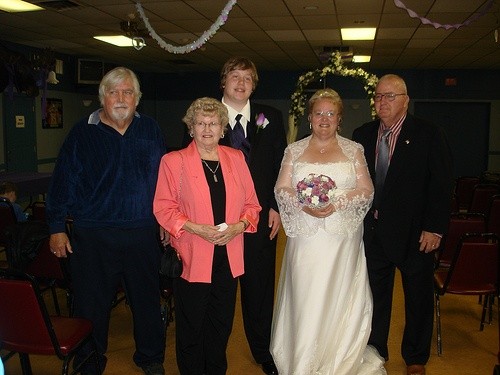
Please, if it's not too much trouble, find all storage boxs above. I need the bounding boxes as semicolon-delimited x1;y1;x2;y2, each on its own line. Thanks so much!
40;97;65;130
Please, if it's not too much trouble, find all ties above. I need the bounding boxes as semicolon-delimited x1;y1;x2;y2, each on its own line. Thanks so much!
231;114;245;149
373;128;392;200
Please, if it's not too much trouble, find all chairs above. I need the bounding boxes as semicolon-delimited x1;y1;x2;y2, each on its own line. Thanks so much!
0;195;174;375
432;170;500;358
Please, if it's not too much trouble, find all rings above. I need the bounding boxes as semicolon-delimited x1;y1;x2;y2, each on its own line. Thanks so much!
432;244;435;247
53;251;56;253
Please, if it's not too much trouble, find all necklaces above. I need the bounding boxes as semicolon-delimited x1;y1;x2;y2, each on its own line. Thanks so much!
201;155;220;183
320;144;328;153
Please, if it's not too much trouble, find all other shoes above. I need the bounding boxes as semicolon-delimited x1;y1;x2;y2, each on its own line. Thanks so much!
143;362;164;375
407;364;425;375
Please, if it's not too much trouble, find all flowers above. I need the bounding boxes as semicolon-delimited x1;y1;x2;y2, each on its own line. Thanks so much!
288;48;380;127
296;172;338;211
254;112;270;134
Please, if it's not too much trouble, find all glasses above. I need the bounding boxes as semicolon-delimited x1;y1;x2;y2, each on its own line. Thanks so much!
311;110;340;118
372;92;407;102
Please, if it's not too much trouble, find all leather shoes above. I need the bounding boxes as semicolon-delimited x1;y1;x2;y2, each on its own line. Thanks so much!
262;360;279;375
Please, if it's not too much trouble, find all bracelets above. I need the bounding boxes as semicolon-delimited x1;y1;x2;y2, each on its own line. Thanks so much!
240;220;247;230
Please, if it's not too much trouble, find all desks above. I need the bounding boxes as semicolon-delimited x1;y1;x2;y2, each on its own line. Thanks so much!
0;169;53;196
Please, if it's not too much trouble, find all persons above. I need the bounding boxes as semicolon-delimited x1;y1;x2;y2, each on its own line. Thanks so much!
152;96;262;375
351;74;453;375
45;67;167;375
0;182;29;222
269;87;389;375
217;57;287;375
